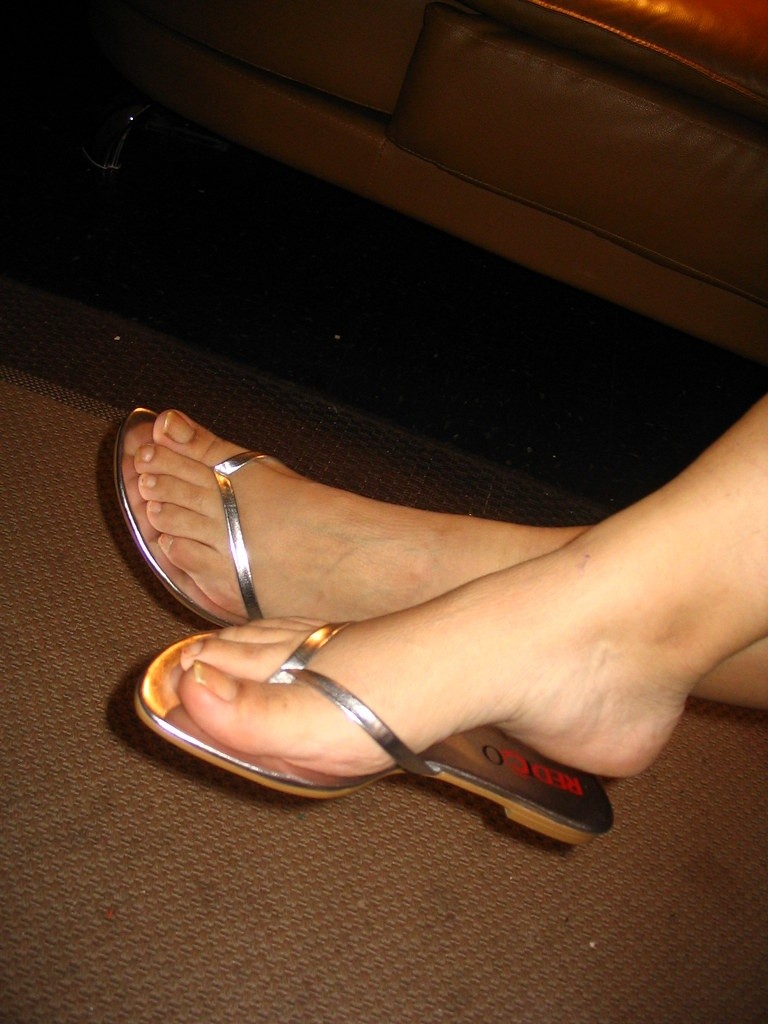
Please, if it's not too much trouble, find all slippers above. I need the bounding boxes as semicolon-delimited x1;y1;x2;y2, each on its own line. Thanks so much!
111;405;268;629
133;615;615;849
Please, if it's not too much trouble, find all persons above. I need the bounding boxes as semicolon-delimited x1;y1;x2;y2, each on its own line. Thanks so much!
110;394;766;846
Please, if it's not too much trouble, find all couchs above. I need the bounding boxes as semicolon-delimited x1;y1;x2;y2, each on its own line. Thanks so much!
77;0;768;366
